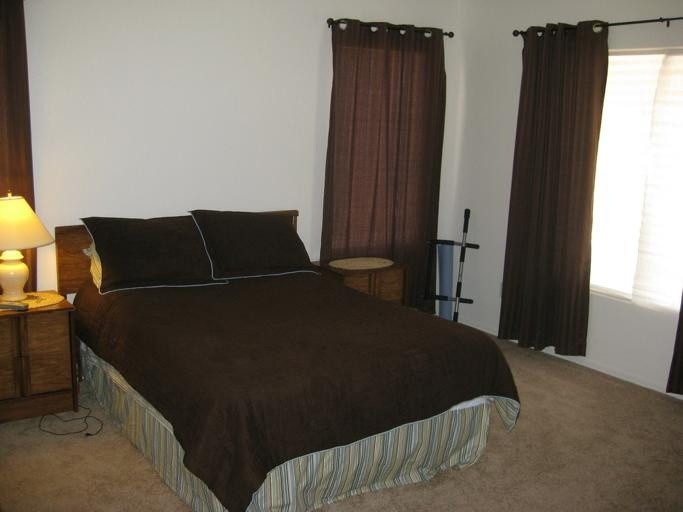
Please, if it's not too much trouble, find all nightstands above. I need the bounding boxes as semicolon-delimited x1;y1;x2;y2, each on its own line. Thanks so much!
322;256;406;305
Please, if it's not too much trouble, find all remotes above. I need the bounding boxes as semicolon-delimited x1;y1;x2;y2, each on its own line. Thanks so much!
0;301;30;311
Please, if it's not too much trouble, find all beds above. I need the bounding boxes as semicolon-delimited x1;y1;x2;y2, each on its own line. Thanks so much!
54;223;522;512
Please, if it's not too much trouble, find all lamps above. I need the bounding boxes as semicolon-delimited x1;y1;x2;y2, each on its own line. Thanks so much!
0;190;56;302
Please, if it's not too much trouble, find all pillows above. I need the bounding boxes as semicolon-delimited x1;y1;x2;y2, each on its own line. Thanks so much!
78;215;229;295
186;208;320;280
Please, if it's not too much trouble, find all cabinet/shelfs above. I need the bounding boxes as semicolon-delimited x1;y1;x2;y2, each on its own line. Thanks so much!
0;290;76;423
